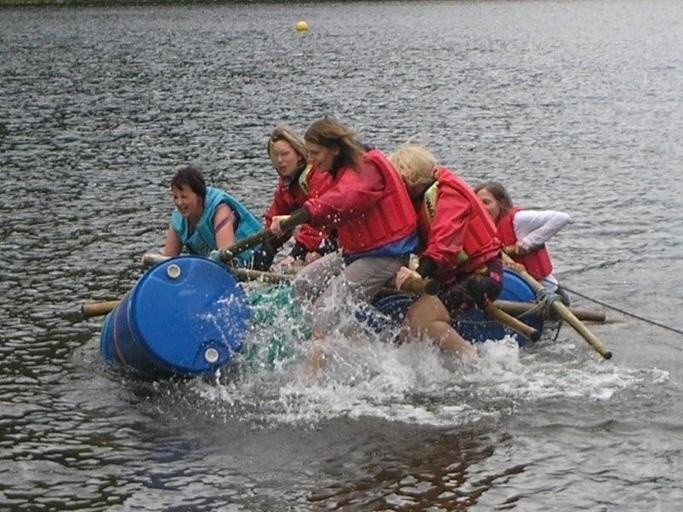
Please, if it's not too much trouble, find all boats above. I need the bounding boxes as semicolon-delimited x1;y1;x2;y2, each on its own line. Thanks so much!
99;270;544;377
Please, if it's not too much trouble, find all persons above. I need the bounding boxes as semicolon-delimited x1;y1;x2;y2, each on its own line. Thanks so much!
272;117;417;373
473;181;571;293
262;128;338;271
384;146;503;355
160;166;276;284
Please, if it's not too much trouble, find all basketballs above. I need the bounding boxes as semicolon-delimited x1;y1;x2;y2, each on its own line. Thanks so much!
297;21;308;31
